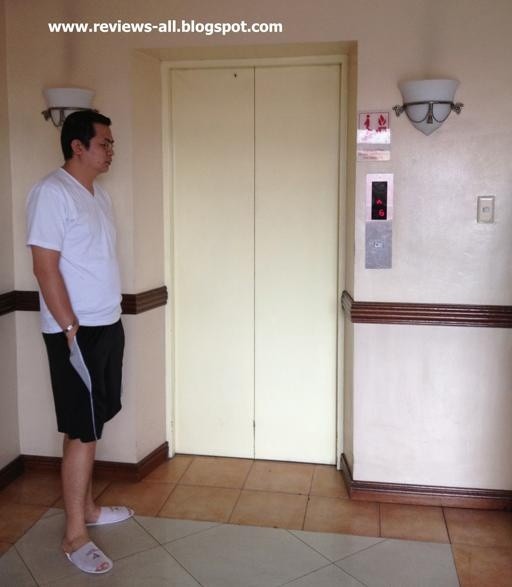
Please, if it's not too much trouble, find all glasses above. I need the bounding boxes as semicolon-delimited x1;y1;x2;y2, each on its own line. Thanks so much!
103;141;114;153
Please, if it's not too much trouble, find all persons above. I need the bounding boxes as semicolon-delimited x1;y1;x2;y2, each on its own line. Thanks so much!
25;110;137;575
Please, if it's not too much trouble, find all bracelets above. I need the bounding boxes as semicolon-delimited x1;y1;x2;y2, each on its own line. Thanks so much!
64;317;78;334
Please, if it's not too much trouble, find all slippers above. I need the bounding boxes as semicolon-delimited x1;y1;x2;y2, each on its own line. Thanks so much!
63;540;114;575
83;504;135;528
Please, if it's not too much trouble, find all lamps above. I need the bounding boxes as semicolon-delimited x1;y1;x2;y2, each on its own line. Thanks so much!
40;87;96;133
394;77;462;136
